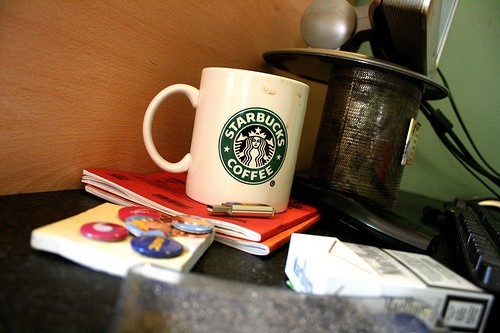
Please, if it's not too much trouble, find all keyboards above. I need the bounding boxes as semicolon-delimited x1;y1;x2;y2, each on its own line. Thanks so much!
446;197;500;295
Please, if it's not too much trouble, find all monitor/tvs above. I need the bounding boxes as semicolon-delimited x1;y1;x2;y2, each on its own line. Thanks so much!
262;0;458;101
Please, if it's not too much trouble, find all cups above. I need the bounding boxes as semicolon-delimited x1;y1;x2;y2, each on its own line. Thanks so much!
142;67;310;213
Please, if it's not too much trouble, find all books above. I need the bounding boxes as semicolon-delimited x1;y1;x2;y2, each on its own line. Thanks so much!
31;166;322;285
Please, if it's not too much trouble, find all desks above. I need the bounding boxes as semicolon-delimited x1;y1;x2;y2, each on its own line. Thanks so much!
0;171;500;333
